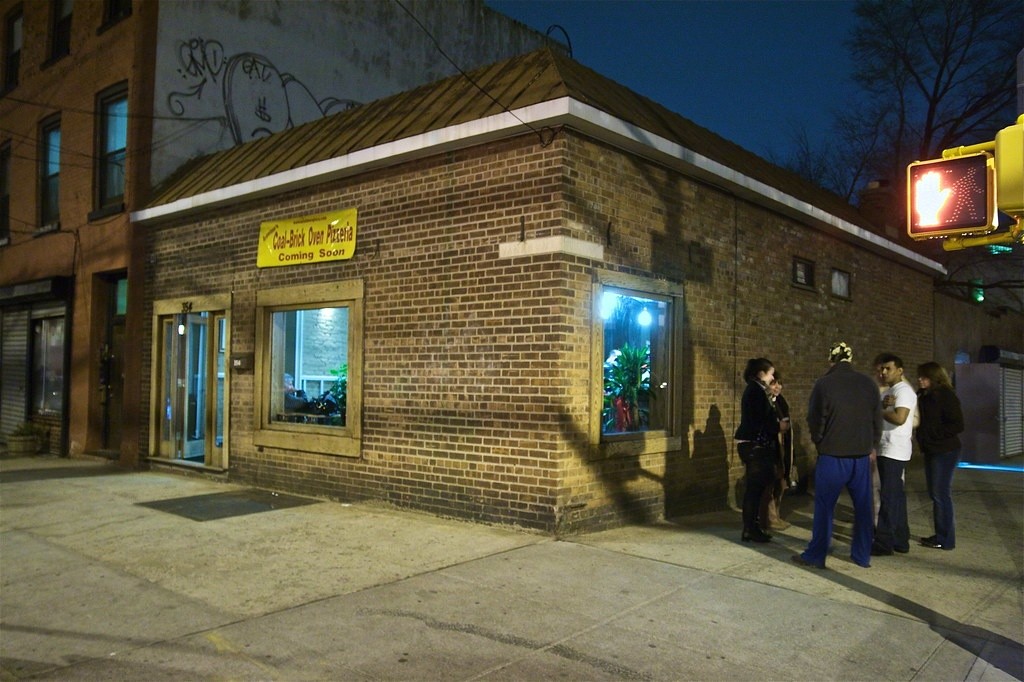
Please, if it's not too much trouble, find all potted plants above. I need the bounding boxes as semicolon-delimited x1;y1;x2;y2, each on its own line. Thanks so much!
7;424;44;458
602;341;657;433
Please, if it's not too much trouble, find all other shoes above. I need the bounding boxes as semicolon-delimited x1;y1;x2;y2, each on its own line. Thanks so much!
921;536;956;549
850;555;871;569
870;540;891;556
791;554;824;569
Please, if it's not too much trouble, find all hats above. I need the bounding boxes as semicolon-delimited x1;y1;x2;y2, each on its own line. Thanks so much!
827;340;852;364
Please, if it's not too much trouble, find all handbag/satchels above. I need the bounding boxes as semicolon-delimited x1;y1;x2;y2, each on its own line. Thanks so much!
749;445;784;478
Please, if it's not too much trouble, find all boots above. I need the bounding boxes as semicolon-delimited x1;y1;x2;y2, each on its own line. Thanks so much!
741;510;772;543
767;500;792;531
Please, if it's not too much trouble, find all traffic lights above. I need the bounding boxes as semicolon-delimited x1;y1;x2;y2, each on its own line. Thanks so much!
907;148;995;237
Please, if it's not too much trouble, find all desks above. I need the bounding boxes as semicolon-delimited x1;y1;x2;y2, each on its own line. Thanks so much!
277;413;341;426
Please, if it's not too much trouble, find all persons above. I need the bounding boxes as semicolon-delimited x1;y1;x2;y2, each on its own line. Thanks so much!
870;356;916;556
284;374;306;421
917;362;963;550
791;341;884;568
767;371;790;529
873;353;921;524
733;358;790;542
